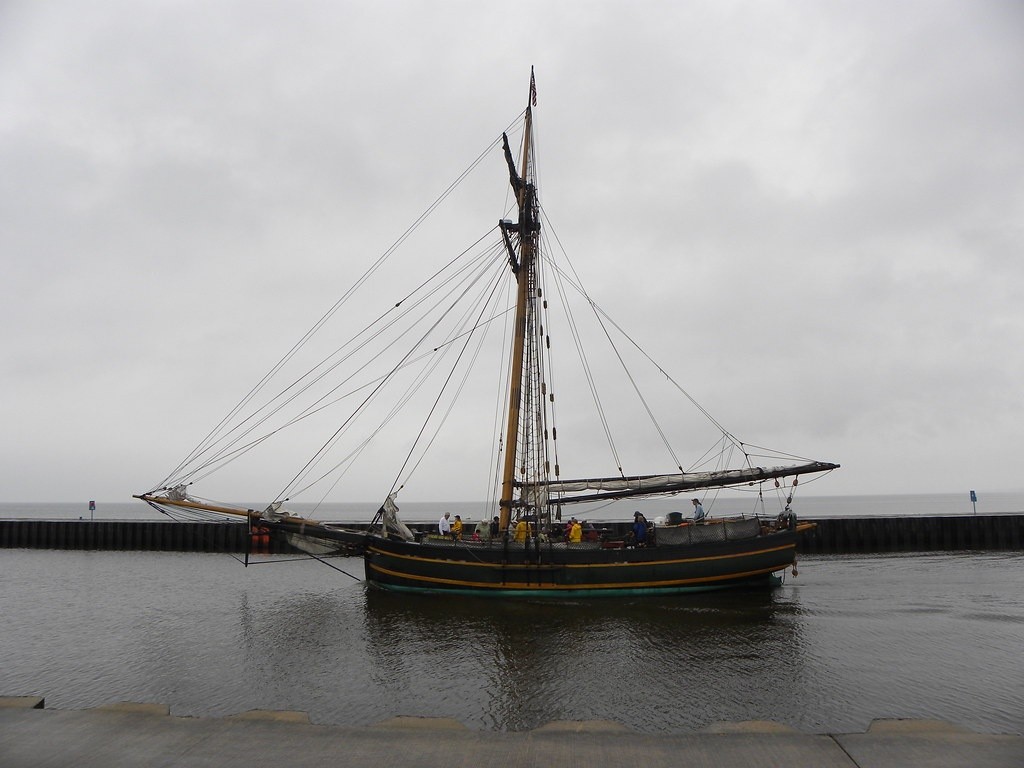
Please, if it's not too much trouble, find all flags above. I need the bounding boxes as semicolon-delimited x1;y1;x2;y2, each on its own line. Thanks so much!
529;70;537;107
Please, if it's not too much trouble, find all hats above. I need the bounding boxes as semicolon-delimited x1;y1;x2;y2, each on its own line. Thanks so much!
476;529;480;533
571;519;577;523
454;515;460;520
554;520;560;524
692;499;699;502
445;512;450;516
481;519;489;523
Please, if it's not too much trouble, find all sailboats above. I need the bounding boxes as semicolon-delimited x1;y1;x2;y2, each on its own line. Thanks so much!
132;60;841;608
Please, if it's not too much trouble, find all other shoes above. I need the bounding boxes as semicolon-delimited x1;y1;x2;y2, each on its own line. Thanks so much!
627;546;635;549
638;543;646;547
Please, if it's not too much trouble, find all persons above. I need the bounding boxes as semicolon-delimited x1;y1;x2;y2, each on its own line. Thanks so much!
631;510;649;549
450;514;463;542
472;515;533;543
439;511;451;536
691;498;705;527
542;518;598;543
